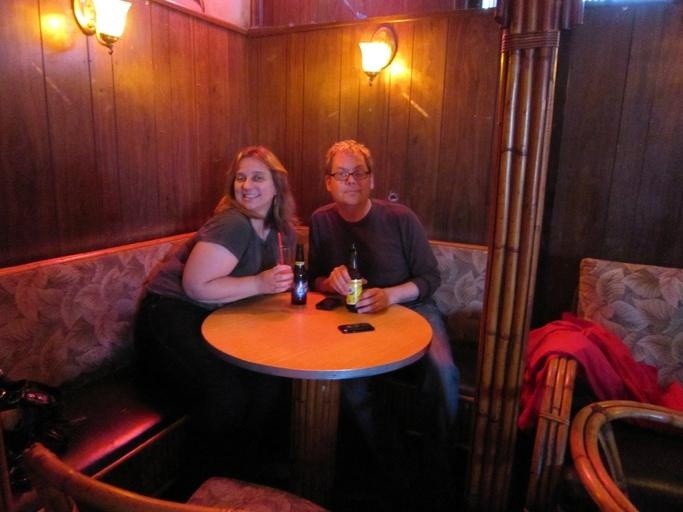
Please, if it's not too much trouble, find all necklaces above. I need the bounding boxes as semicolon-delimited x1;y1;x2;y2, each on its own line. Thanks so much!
348;241;358;270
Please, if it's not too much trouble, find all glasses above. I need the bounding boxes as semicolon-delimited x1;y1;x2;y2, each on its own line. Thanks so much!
330;171;369;180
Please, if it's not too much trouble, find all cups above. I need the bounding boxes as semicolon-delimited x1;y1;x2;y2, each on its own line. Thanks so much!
273;245;291;266
346;240;362;312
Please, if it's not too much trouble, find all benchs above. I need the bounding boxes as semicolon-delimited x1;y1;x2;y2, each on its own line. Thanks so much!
0;223;288;500
287;222;487;411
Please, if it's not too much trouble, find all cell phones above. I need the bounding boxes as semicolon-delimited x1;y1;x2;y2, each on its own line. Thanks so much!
338;323;375;334
316;296;341;310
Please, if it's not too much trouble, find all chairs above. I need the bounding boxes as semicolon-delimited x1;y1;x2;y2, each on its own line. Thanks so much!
567;397;682;511
520;254;682;512
19;440;336;511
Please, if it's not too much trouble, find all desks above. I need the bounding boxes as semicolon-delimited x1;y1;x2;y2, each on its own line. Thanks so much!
198;288;434;509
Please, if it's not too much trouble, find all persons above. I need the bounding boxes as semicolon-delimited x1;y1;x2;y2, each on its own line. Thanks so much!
308;141;474;510
132;146;299;489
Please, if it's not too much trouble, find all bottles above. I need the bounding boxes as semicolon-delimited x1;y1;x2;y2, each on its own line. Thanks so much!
292;242;309;305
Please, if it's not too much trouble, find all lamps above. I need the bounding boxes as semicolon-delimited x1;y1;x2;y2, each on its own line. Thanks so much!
67;0;132;57
354;21;399;87
